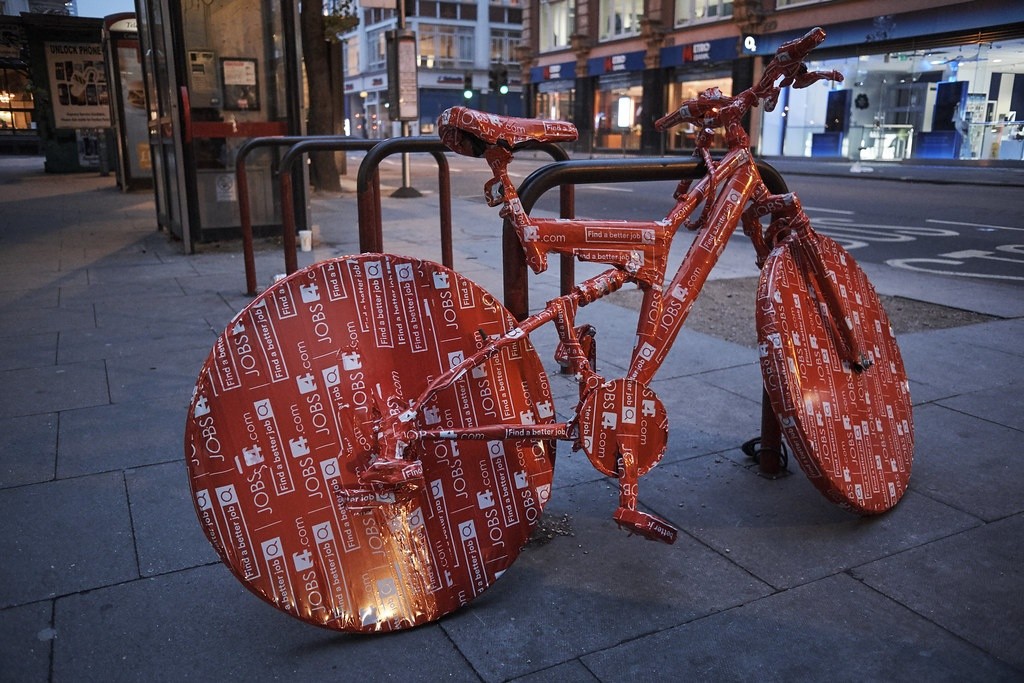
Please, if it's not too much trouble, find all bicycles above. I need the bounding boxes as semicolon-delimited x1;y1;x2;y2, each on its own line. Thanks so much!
183;25;917;628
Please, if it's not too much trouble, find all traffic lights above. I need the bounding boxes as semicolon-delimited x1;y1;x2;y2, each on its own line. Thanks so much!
488;69;498;94
464;77;473;102
497;66;510;96
